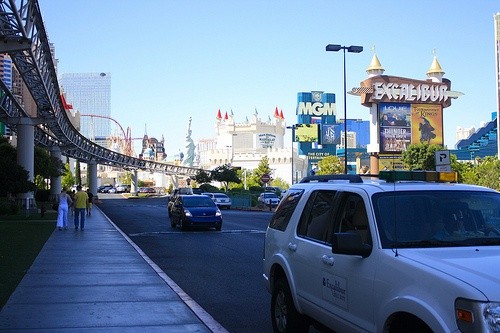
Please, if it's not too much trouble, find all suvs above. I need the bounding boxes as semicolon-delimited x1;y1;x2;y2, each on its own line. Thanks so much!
264;170;499;333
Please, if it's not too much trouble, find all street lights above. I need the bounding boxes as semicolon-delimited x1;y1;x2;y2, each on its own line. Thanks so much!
325;43;365;173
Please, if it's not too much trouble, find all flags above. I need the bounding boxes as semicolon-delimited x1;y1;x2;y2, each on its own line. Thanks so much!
216;108;222;121
279;109;285;119
224;111;229;121
273;106;280;119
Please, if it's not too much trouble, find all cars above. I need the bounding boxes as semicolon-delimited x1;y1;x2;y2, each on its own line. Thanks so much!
170;195;223;230
138;187;155;193
167;187;205;218
258;193;281;208
264;186;286;194
98;184;131;194
202;193;234;209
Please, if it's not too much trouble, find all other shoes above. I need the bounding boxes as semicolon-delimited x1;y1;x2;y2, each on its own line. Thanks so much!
89;211;91;216
75;227;78;230
81;228;84;231
64;227;67;230
59;227;62;231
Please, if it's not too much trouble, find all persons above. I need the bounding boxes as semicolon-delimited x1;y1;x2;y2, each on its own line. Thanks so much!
56;186;74;231
171;189;179;202
71;188;93;216
72;185;89;233
